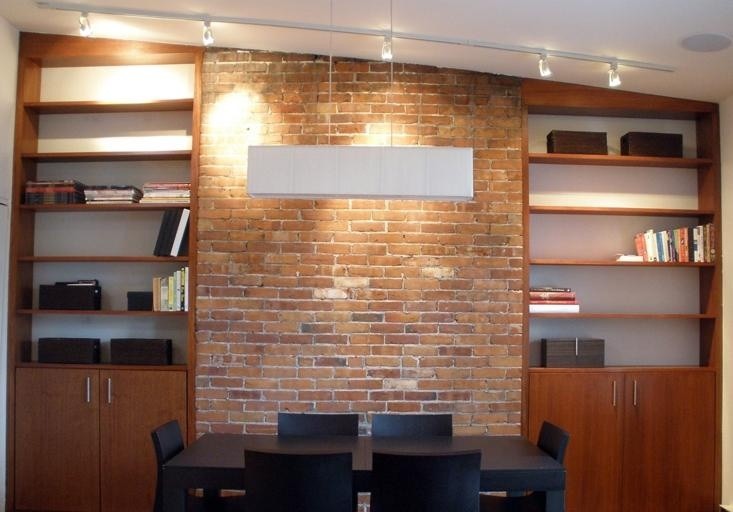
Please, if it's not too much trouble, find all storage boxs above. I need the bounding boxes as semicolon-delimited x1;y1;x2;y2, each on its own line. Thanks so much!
110;338;172;366
127;291;153;311
620;131;682;157
38;338;99;364
546;129;608;155
541;337;604;368
39;280;100;311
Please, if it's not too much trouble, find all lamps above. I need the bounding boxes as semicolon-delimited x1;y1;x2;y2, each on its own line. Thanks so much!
36;0;674;86
246;1;474;203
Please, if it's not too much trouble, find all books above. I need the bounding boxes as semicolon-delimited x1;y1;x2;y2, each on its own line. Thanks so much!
635;222;714;262
153;267;188;312
153;209;190;257
528;287;580;313
23;180;192;203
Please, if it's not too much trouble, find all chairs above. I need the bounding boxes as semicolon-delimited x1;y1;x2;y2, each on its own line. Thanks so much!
370;413;453;439
151;419;185;511
243;448;359;512
501;420;569;512
276;413;359;438
369;449;482;512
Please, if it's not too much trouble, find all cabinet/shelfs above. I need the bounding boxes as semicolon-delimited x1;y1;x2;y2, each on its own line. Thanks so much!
523;75;722;511
6;32;200;511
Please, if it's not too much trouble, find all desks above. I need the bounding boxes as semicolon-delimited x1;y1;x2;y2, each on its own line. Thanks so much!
160;434;565;512
